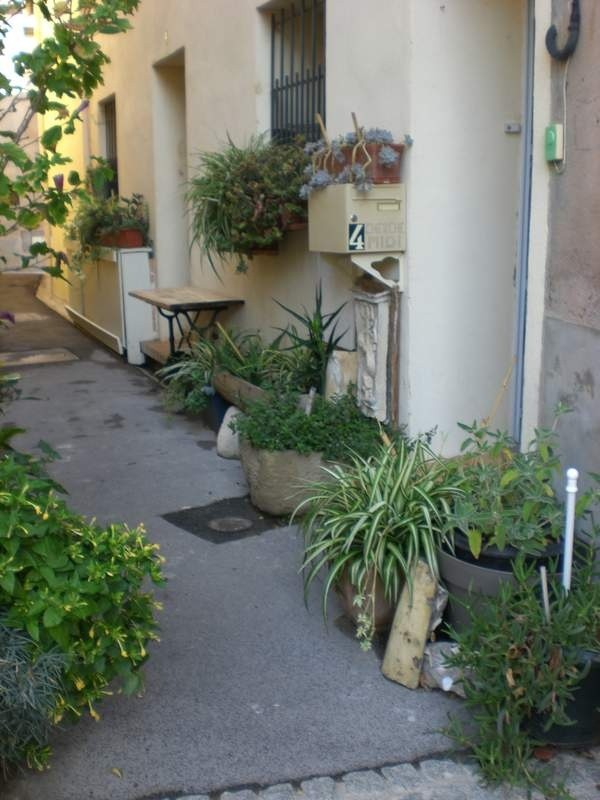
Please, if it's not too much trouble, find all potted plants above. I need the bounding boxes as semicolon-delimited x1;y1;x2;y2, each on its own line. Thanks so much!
65;193;148;247
147;278;600;800
175;137;311;256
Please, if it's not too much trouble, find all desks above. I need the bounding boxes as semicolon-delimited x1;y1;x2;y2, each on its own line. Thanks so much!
128;288;246;386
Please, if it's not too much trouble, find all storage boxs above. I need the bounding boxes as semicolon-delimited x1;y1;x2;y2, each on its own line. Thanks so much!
309;185;406;252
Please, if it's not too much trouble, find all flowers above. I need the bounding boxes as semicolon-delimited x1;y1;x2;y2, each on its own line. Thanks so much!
300;127;412;199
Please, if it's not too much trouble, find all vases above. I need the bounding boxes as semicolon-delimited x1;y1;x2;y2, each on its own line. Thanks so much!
316;144;405;185
